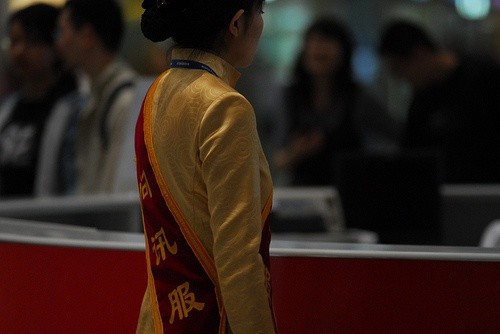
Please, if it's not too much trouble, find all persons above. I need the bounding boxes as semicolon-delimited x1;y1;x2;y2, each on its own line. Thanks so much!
335;152;448;247
126;0;277;334
277;20;363;186
64;0;147;196
2;3;79;198
376;15;500;181
